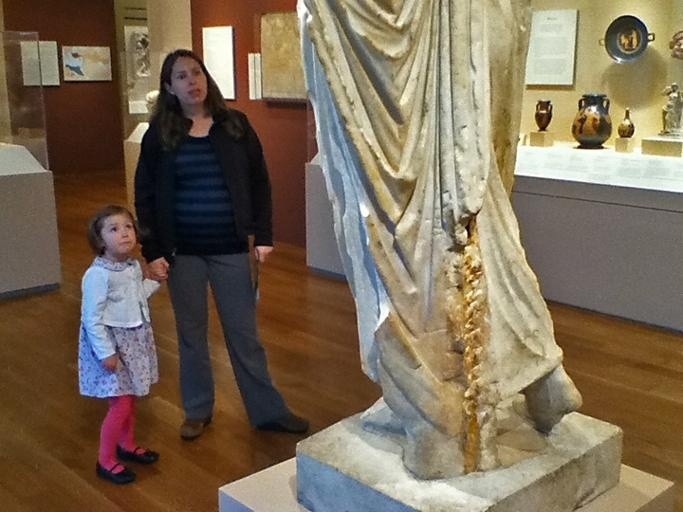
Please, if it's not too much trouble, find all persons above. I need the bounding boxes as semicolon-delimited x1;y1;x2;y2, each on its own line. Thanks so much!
134;49;308;442
294;1;584;477
77;204;171;484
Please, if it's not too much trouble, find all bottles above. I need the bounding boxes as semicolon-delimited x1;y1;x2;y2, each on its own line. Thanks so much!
617;106;634;137
572;93;614;149
535;98;553;132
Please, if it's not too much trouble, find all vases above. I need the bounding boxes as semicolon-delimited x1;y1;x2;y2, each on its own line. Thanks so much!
533;94;635;150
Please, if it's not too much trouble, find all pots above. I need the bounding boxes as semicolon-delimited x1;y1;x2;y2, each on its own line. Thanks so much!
597;14;654;64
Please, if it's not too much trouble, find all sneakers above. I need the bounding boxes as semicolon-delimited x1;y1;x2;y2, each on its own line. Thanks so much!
180;416;211;440
255;415;309;434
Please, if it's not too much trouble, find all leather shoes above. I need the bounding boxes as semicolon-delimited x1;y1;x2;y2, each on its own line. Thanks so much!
118;446;160;465
95;461;135;484
